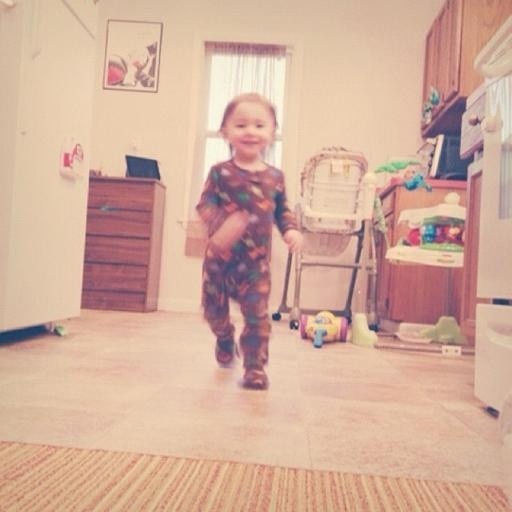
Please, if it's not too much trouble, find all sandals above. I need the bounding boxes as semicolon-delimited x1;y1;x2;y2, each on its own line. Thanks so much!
214;333;235;363
242;364;268;389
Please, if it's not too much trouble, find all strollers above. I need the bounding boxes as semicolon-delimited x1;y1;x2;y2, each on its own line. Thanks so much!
272;145;378;332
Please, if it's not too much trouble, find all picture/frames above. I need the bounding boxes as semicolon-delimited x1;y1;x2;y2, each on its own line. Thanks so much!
103;18;164;93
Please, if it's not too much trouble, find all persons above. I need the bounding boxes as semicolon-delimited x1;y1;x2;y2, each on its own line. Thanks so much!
196;93;304;391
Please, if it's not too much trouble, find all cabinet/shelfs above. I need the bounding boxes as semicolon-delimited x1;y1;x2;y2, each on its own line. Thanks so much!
418;0;511;138
80;174;165;314
365;181;469;334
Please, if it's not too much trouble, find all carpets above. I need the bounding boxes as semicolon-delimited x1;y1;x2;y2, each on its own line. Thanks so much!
0;441;510;511
